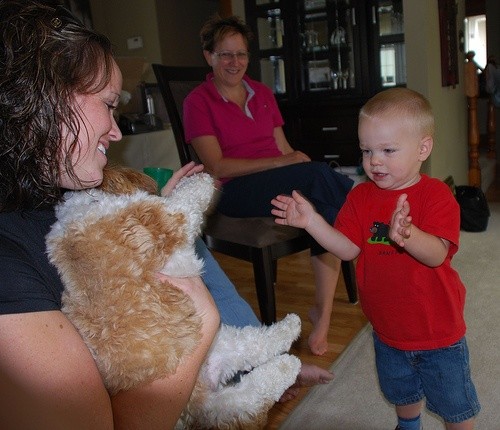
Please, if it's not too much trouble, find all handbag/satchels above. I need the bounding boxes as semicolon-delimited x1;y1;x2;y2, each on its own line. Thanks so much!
455;185;490;232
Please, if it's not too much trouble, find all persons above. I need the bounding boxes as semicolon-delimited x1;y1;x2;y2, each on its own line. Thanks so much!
183;13;366;355
0;1;334;430
271;88;482;430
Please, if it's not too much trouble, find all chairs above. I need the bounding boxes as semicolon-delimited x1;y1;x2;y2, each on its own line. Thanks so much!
151;64;358;327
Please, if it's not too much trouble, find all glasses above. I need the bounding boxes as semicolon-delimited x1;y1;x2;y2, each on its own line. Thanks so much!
213;49;249;60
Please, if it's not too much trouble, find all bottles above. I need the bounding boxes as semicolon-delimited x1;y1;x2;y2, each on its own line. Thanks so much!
146;93;155;114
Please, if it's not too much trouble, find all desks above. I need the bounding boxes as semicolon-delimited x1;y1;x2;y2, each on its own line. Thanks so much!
110;121;181;172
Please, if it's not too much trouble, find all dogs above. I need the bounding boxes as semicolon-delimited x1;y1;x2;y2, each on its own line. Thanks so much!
42;157;304;430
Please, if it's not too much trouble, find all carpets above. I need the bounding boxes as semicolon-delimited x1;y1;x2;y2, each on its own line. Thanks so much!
278;202;500;430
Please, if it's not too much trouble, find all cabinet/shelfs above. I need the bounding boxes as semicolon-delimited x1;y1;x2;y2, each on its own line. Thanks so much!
244;0;406;166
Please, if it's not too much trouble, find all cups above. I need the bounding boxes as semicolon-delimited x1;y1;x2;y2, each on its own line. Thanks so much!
144;167;173;196
